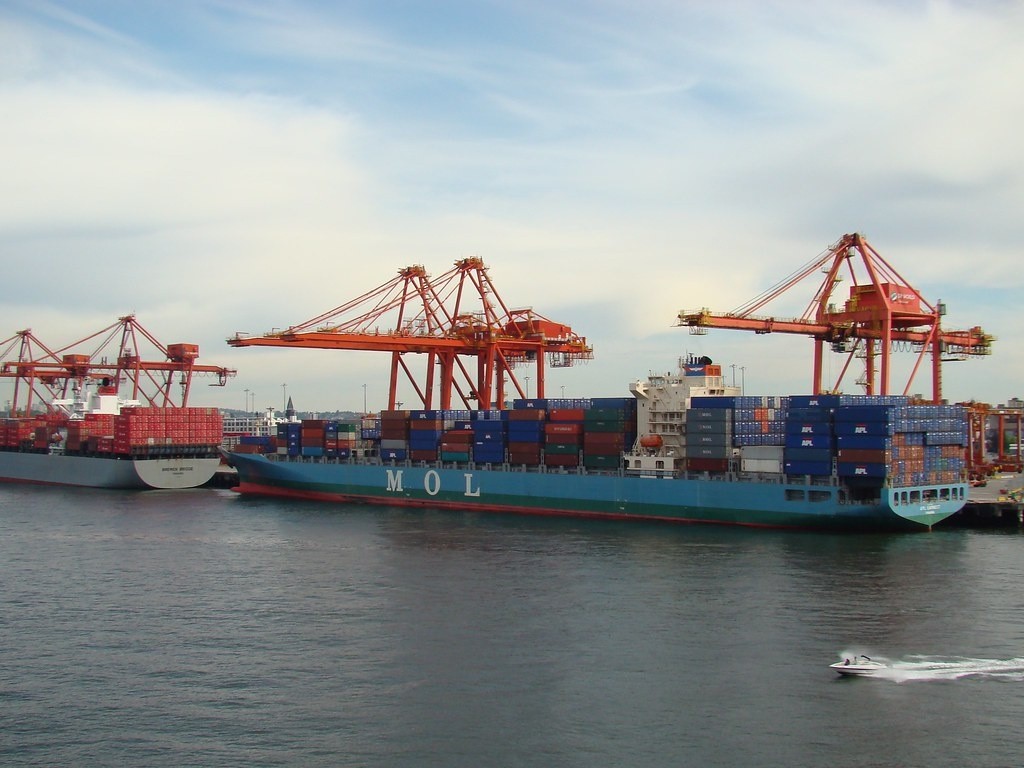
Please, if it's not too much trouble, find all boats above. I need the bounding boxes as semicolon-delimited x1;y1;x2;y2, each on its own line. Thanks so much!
216;445;969;531
0;442;223;489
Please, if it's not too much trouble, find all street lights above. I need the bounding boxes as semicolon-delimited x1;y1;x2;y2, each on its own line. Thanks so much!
729;364;737;388
362;384;367;415
244;389;250;414
280;383;288;423
266;406;274;434
250;393;256;418
739;366;746;396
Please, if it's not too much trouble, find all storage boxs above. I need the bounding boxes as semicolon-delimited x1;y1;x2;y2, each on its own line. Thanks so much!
239;398;971;502
0;406;224;454
850;283;920;313
456;320;571;339
63;354;90;365
167;343;199;358
683;363;721;376
